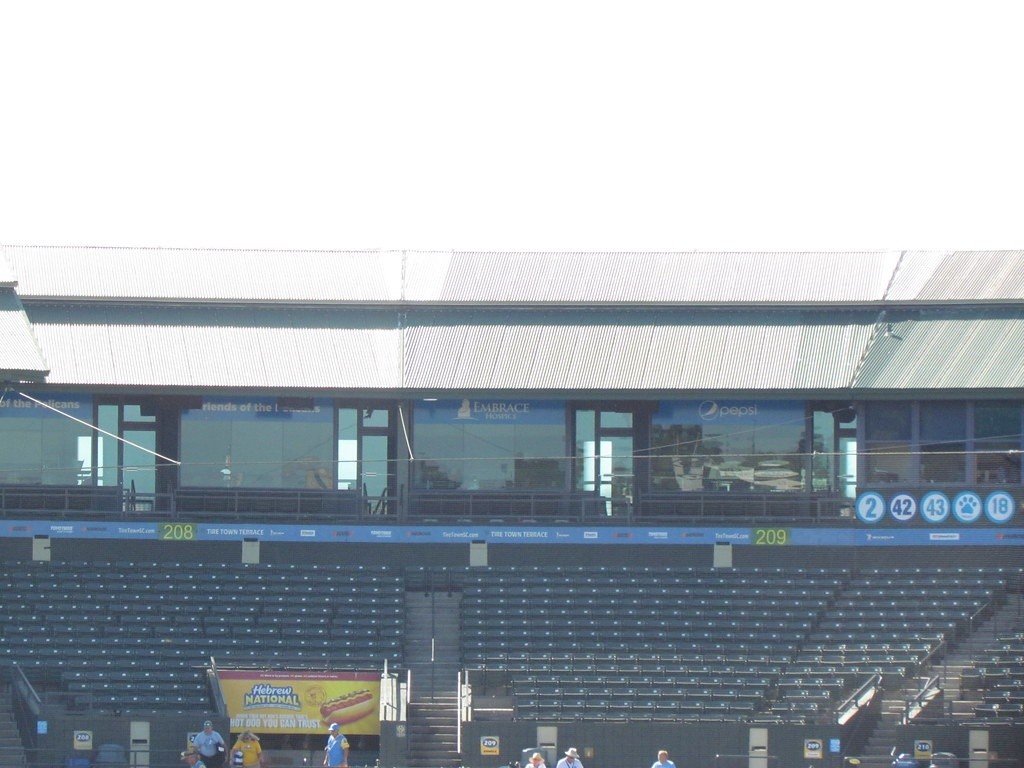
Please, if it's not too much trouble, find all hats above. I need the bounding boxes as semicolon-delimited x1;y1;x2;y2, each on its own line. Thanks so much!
204;720;212;725
529;753;544;764
565;748;579;758
180;751;196;760
328;723;339;730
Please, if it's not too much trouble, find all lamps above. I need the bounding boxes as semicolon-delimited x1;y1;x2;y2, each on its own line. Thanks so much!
884;324;894;336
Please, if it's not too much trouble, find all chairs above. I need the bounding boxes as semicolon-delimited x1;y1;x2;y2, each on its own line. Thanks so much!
428;566;1024;726
0;560;427;719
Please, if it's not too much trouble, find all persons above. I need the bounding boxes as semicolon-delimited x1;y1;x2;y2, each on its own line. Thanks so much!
182;750;205;768
650;751;676;768
525;753;546;768
230;731;264;768
555;748;583;768
323;723;348;768
193;721;228;768
